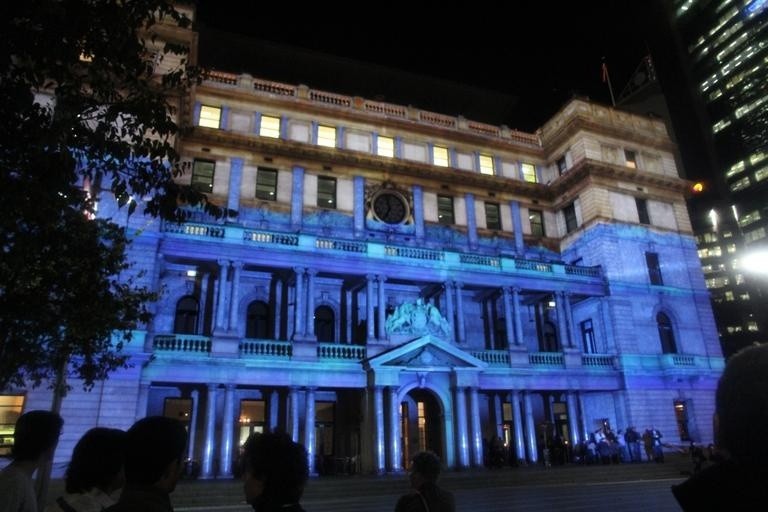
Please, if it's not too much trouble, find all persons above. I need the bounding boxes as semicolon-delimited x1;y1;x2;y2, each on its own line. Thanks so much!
241;425;309;511
49;427;127;511
395;449;457;511
688;440;707;471
707;443;719;462
1;409;64;511
104;415;191;512
537;424;663;464
480;435;516;470
671;345;768;512
